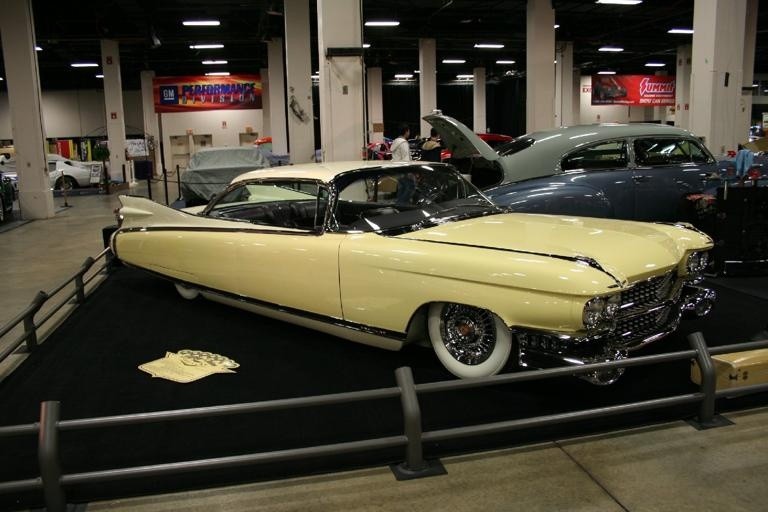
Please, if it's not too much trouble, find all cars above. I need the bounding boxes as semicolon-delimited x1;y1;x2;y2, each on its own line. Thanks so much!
0;153;107;200
420;108;737;227
109;157;721;387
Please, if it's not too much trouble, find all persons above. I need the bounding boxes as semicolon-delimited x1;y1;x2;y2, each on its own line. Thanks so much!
390;124;442;162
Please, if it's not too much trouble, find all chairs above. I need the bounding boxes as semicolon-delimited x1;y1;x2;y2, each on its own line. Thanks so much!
221;197;339;229
